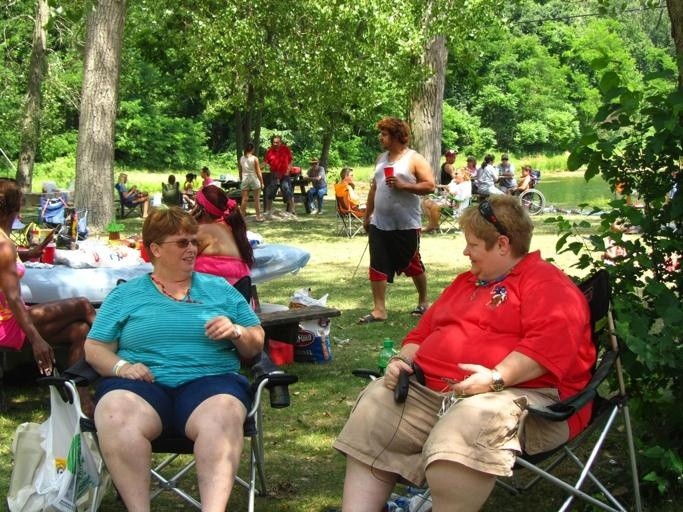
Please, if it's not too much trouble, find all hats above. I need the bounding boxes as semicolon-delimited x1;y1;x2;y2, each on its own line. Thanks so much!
445;150;458;157
309;156;321;163
501;154;509;161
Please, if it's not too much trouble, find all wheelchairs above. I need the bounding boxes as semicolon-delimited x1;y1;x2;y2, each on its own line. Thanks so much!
507;169;546;216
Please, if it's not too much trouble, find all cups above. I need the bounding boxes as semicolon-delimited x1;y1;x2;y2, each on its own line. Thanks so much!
42;244;57;262
384;167;395;179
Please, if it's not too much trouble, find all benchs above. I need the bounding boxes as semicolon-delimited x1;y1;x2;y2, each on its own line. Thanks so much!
252;304;342;371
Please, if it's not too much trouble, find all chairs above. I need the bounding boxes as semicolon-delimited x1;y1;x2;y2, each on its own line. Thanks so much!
115;159;369;241
424;175;514;239
8;217;29;247
352;270;644;511
52;274;299;512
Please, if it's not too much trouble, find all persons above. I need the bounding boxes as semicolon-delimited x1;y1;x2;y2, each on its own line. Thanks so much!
85;207;266;512
332;192;597;512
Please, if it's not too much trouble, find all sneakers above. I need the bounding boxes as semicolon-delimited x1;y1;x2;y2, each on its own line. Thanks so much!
256;209;323;222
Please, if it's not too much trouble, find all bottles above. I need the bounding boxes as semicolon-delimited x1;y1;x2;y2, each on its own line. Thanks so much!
29;230;41;263
269;370;290;407
376;339;399;375
385;486;426;512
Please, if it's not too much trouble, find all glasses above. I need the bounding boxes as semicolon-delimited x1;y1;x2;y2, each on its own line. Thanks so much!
161;238;199;247
477;194;505;233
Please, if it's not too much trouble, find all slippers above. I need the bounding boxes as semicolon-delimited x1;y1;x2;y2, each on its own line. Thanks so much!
411;306;430;317
357;313;386;323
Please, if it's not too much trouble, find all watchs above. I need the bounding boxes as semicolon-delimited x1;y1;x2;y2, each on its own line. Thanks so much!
490;367;505;393
